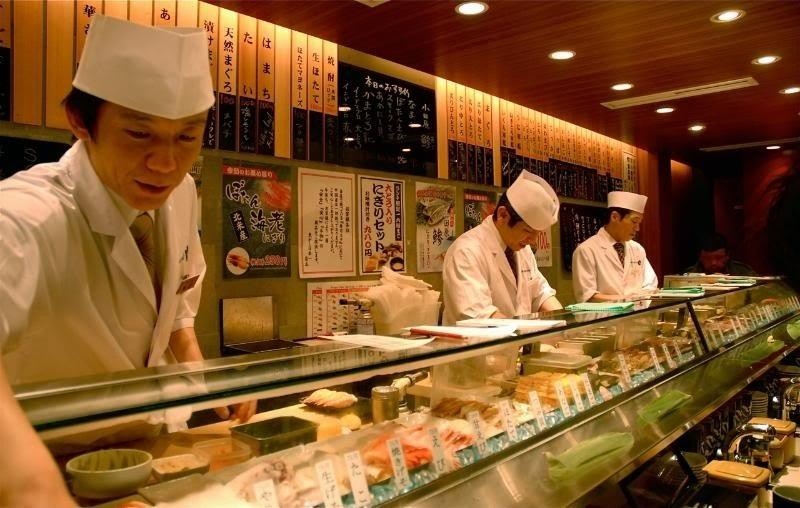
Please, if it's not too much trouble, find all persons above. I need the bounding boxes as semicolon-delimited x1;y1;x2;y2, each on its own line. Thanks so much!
0;13;259;508
427;168;566;409
572;190;658;303
684;238;760;278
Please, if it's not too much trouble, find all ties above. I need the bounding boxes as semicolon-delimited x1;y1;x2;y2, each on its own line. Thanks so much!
614;243;624;269
505;247;517;282
130;215;161;306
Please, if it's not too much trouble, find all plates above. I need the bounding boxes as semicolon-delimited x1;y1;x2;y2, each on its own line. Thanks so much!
746;390;768;417
89;308;743;508
226;247;250;276
774;365;800;375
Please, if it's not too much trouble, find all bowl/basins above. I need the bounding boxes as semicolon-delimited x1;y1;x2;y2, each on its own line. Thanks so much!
390;257;404;271
66;448;154;499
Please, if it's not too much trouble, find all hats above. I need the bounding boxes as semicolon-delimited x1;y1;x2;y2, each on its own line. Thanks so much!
607;192;647;214
506;170;559;230
72;15;216;120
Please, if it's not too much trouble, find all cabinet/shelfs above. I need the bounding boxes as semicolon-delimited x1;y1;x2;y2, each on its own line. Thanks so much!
5;276;800;508
